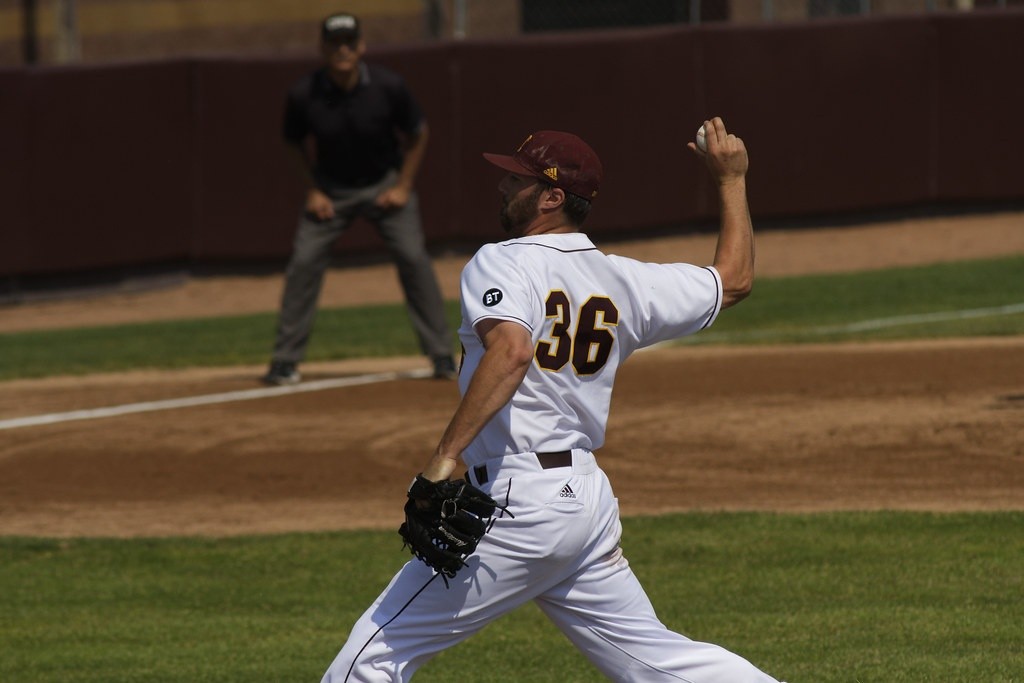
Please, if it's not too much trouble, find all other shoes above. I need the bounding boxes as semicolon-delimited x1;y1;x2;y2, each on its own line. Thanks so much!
262;361;301;387
432;354;458;380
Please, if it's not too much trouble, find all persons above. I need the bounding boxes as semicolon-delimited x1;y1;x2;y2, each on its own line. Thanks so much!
319;116;783;683
257;12;456;385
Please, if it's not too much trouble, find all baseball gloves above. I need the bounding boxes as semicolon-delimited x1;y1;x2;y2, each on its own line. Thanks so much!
397;472;516;593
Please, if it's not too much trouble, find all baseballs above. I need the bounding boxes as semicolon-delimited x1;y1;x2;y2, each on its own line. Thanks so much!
696;123;727;153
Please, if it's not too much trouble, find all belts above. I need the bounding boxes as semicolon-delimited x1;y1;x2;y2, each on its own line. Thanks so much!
464;450;572;486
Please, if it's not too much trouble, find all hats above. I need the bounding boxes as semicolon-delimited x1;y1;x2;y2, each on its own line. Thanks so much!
321;13;359;42
483;131;601;201
